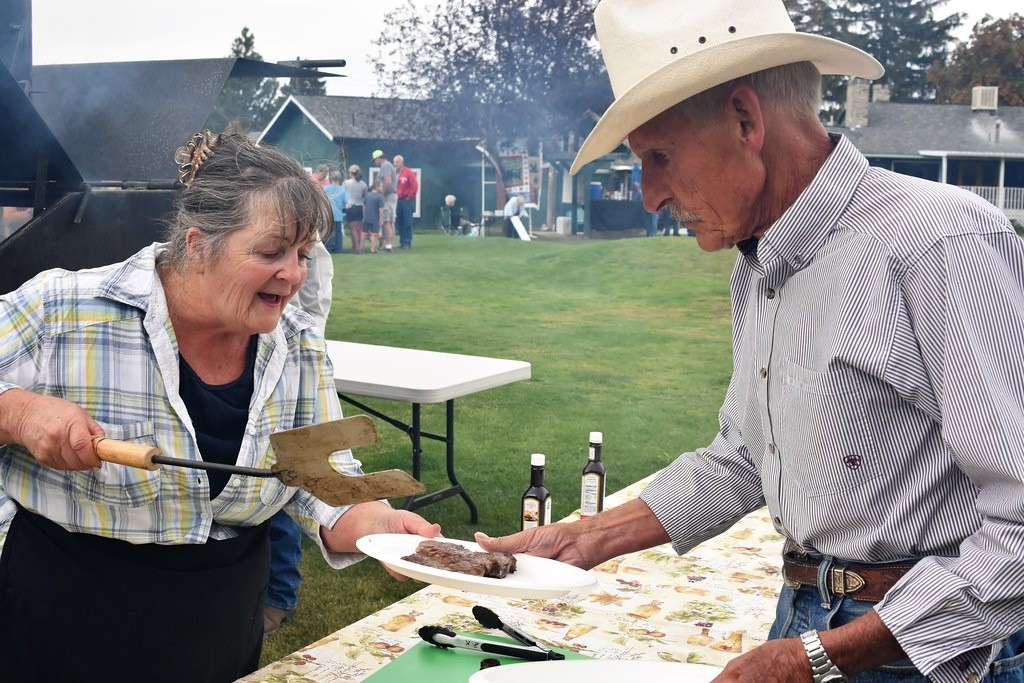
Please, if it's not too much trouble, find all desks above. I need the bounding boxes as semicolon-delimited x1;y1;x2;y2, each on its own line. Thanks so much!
326;339;532;524
582;200;664;230
235;467;787;683
482;215;513;237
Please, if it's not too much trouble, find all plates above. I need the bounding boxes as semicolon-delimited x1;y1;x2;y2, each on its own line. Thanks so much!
468;659;725;683
355;532;597;599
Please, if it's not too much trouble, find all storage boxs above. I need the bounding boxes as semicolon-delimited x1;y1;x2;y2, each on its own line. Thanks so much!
484;211;492;215
590;182;603;200
494;210;503;216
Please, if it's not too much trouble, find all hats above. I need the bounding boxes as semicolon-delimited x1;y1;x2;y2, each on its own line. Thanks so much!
566;0;885;175
348;164;361;172
369;149;385;162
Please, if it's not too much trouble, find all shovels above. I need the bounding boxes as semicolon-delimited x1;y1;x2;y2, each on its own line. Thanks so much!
89;415;427;508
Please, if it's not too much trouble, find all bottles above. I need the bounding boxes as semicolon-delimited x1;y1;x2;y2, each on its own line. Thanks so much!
521;453;552;536
581;432;606;518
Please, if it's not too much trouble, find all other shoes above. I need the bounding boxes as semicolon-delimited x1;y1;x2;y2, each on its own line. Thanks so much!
377;246;393;252
262;603;298;635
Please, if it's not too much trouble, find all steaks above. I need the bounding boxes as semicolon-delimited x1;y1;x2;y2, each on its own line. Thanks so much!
400;540;517;578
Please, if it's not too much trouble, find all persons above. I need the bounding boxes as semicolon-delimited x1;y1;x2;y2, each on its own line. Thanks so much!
474;0;1023;683
1;127;447;682
440;195;474;239
503;194;531;238
632;181;681;237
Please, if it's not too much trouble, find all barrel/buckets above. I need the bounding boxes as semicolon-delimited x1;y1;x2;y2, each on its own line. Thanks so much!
590;181;602;200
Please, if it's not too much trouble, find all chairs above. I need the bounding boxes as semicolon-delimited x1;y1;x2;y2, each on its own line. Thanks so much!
441;207;469;235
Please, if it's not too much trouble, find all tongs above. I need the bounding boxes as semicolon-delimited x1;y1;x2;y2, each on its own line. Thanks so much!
417;605;565;661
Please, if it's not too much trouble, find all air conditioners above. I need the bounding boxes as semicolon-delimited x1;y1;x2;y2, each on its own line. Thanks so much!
972;86;998;109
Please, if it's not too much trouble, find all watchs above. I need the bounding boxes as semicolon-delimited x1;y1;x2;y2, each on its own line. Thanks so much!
800;629;849;683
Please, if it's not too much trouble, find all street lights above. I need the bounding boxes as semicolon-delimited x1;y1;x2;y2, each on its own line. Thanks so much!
481;143;488;237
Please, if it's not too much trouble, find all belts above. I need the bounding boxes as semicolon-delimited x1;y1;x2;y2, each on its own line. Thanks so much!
781;542;916;602
